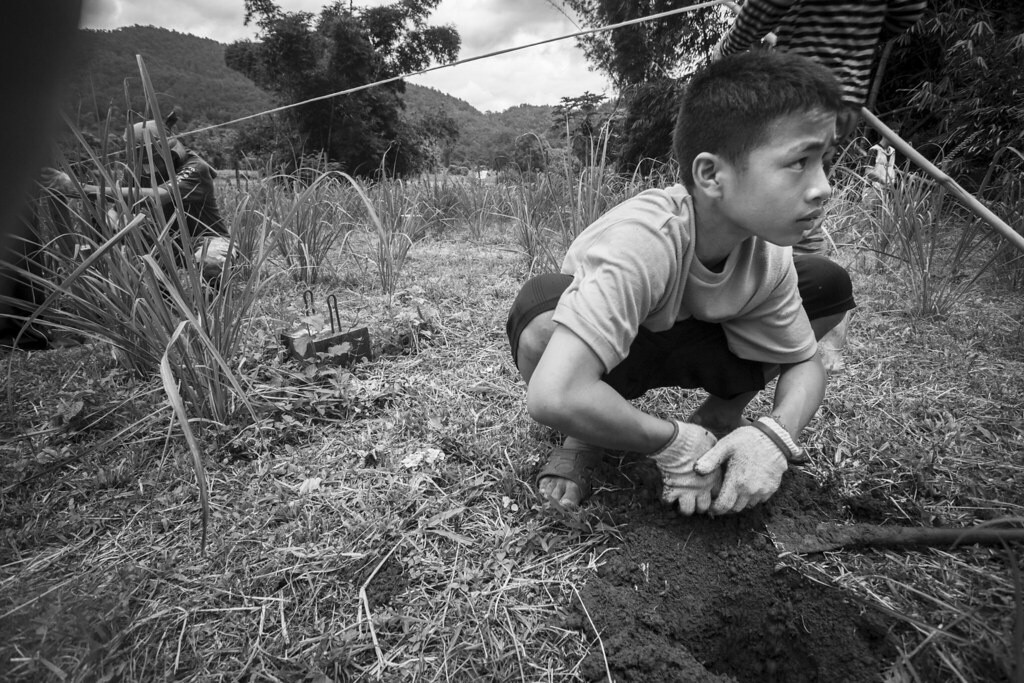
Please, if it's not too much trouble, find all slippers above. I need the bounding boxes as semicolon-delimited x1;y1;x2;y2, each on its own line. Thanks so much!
534;441;602;513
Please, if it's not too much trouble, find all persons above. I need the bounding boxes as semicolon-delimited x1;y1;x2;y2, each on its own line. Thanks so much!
504;52;856;521
709;1;926;374
861;122;901;216
34;121;237;298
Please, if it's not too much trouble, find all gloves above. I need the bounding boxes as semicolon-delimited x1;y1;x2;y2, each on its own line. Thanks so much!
647;414;723;516
694;416;800;517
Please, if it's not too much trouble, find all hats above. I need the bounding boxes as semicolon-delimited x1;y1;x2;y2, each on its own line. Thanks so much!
123;120;188;162
